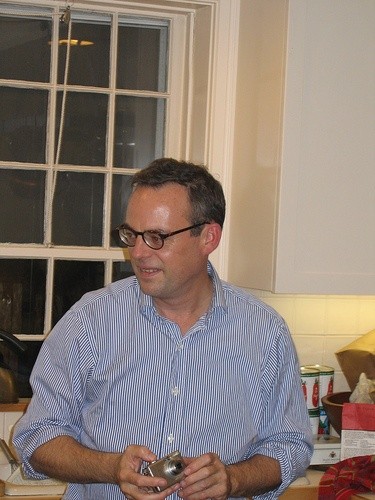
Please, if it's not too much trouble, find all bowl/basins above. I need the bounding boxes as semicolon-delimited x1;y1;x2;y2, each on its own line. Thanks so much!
321;391;352;436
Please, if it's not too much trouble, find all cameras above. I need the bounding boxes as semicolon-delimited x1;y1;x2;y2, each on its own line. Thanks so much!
141;450;187;493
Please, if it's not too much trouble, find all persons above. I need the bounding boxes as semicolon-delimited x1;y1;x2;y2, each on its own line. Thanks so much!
10;156;316;500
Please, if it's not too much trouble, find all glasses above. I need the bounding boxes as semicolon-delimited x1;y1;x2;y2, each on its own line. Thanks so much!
115;221;211;251
